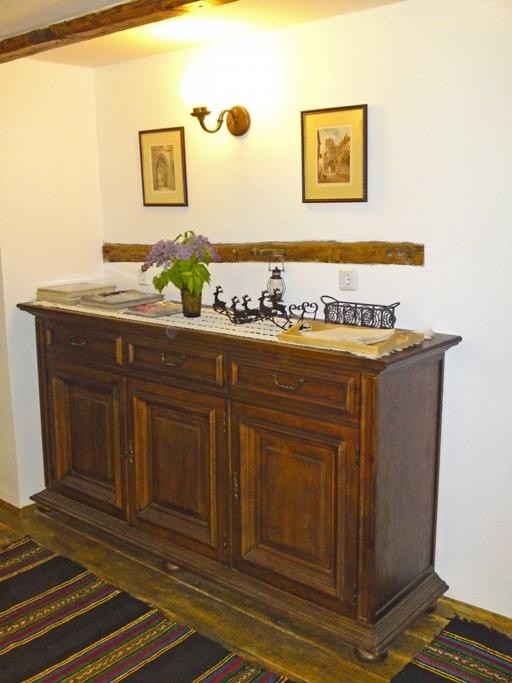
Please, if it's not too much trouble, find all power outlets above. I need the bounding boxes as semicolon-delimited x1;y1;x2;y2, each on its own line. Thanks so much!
339;268;358;291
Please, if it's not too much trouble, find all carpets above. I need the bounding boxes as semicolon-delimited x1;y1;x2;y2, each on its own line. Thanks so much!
0;533;510;682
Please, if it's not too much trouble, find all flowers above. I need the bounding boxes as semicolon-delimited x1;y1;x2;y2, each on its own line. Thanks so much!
140;230;222;297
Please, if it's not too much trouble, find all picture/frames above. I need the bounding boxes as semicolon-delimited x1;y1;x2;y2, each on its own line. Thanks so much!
299;103;371;204
138;127;190;208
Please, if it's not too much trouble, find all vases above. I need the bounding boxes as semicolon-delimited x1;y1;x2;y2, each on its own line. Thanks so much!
180;286;201;317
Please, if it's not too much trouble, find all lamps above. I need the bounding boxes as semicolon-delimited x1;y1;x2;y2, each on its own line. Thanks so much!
266;255;287;303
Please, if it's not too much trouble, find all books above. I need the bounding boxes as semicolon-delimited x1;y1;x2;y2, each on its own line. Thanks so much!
300;327;395;344
35;283;183;317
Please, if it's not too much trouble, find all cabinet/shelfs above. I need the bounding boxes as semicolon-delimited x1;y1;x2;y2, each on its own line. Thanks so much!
16;292;462;664
